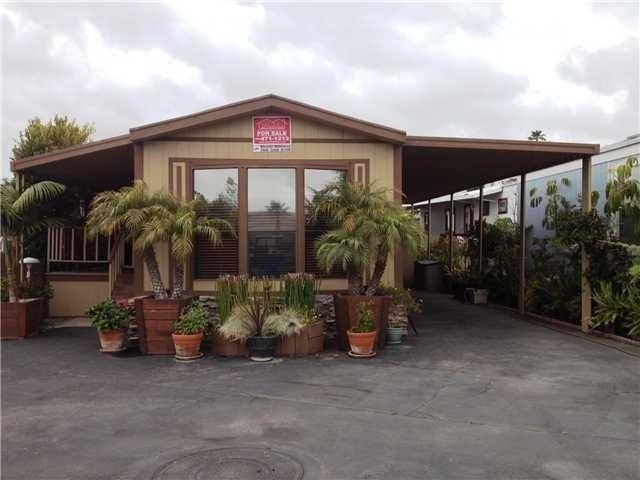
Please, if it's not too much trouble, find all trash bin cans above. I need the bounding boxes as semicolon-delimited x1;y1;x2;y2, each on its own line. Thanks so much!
415;259;443;294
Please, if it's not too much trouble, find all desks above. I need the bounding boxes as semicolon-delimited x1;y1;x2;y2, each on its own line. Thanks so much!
414;259;440;291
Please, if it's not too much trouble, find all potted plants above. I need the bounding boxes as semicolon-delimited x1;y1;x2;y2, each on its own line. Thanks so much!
85;179;236;353
343;298;380;358
84;297;130;353
203;268;328;362
303;177;427;352
0;180;79;342
168;301;209;359
